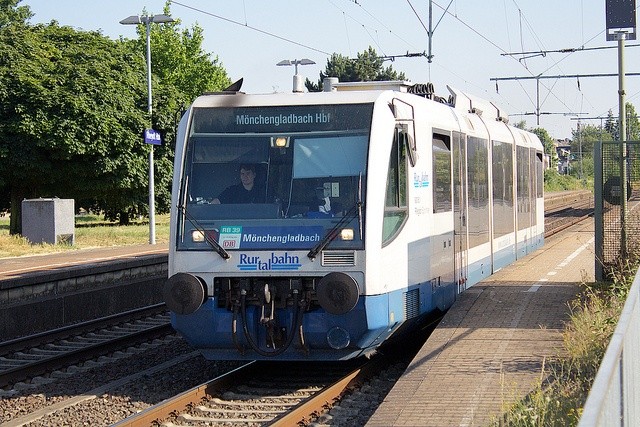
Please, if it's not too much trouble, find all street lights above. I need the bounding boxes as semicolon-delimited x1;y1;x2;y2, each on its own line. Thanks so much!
276;58;317;92
119;14;177;245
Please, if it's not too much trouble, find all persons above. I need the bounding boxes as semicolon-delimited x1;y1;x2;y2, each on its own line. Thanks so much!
210;164;266;204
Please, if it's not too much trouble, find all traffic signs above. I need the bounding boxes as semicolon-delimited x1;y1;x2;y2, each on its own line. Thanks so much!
145;129;161;145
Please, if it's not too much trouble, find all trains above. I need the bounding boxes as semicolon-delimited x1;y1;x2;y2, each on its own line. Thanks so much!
163;78;545;361
